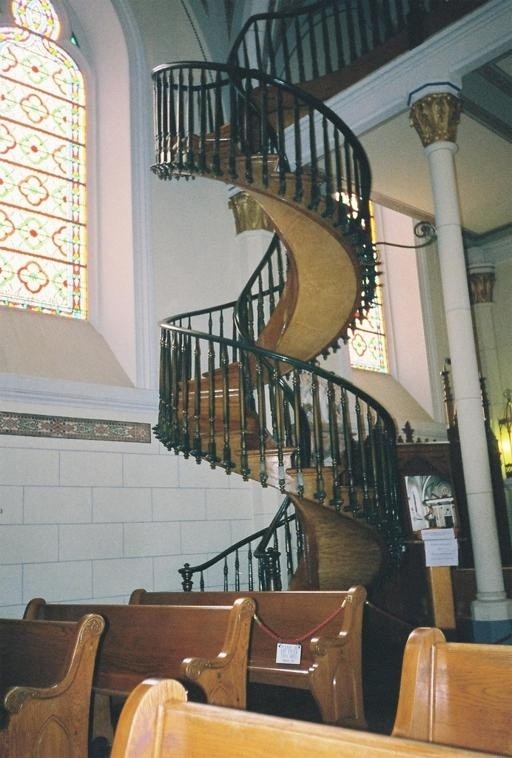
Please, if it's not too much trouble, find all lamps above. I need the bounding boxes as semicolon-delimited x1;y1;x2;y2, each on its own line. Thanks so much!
496;418;512;480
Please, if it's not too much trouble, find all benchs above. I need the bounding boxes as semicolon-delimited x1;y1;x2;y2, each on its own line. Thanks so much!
0;585;512;758
